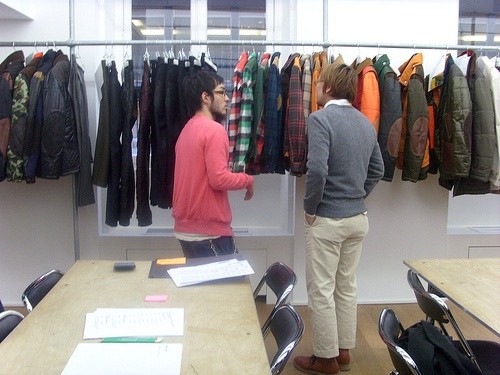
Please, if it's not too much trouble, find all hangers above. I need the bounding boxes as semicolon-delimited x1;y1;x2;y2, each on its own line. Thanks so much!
11;39;210;60
241;40;500;61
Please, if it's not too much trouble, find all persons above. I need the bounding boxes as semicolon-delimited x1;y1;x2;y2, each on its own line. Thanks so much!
171;71;253;262
292;61;385;375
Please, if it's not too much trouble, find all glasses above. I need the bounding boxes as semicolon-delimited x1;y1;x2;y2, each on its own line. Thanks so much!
213;89;227;98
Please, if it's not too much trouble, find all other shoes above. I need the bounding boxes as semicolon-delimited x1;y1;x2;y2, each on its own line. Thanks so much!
294;354;340;375
337;349;350;371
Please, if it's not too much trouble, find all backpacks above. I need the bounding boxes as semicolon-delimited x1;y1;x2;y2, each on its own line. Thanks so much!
399;320;477;375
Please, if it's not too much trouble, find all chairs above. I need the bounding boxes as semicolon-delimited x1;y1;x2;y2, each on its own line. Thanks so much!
0;261;305;375
376;269;500;375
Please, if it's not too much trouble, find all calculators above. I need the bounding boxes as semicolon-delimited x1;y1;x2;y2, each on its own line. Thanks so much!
113;259;137;270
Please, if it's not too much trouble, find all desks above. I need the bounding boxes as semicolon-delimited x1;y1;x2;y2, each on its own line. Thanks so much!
403;257;500;336
0;258;273;375
